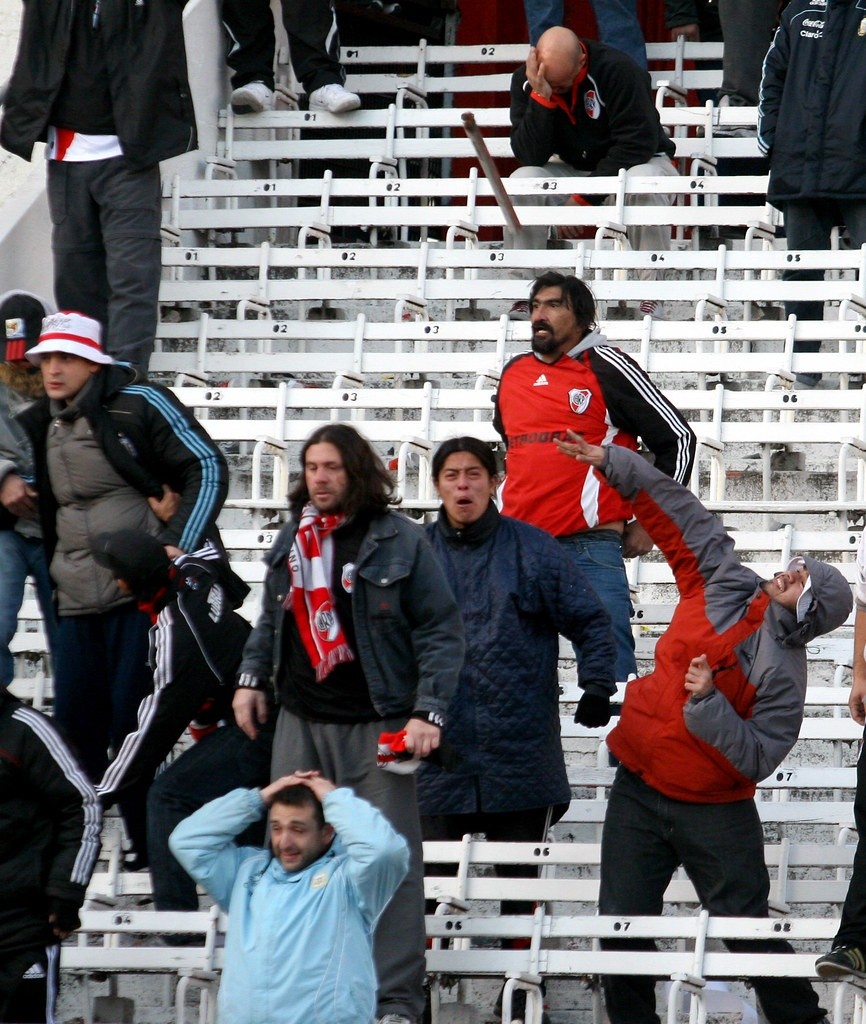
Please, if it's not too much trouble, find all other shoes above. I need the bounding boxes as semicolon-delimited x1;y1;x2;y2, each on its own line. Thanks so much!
492;983;552;1024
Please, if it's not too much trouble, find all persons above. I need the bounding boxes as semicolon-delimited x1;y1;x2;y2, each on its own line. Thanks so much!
0;685;104;1024
0;291;226;873
527;0;651;76
659;0;866;393
488;270;698;770
816;521;866;981
411;436;618;1024
497;26;678;320
550;427;830;1024
170;769;410;1023
82;478;276;947
217;0;363;113
0;0;200;383
230;424;468;1024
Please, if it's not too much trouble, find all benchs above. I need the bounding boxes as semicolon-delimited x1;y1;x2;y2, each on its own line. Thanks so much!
8;32;866;1024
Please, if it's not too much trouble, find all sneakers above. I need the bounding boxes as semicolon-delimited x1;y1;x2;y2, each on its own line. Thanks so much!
308;83;361;114
814;942;866;979
508;301;533;321
635;300;665;320
229;79;273;114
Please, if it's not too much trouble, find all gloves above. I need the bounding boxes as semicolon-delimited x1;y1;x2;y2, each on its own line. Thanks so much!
573;691;613;729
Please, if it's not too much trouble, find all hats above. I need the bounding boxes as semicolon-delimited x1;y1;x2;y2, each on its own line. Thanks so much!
23;309;117;367
90;528;170;583
0;288;46;361
786;556;818;624
